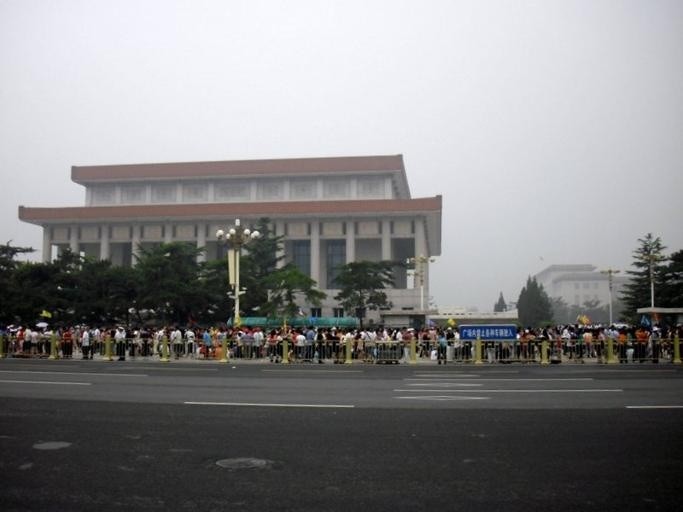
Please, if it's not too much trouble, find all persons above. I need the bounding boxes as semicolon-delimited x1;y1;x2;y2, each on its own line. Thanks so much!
1;316;683;365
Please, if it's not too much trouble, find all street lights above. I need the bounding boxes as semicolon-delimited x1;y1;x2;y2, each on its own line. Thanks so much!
599;267;619;326
409;255;435;312
214;217;260;324
633;249;668;308
